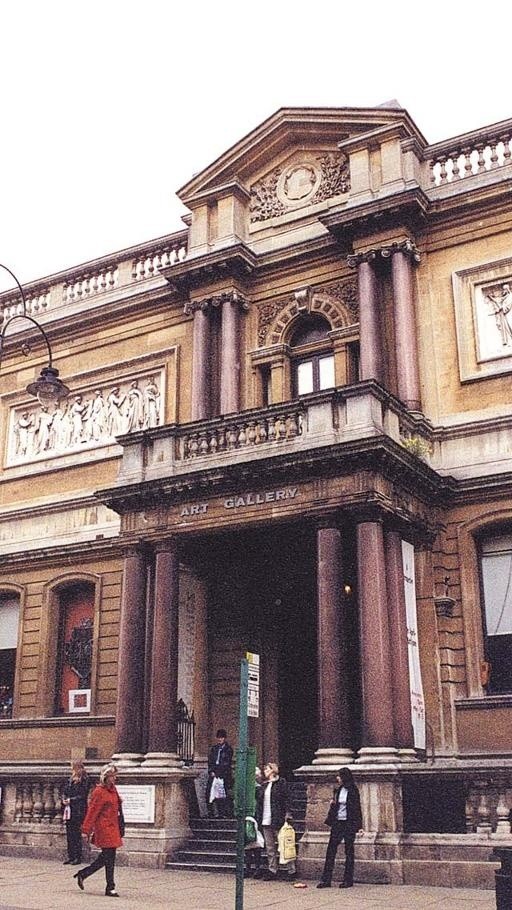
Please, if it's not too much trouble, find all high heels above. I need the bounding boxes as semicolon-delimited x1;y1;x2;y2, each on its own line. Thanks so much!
74;873;84;889
105;890;119;896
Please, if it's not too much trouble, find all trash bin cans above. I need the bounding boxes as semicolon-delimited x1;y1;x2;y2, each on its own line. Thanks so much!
492;845;512;910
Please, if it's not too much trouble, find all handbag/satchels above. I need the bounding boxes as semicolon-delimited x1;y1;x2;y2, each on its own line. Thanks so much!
214;777;226;799
277;822;296;864
118;806;124;837
324;803;336;826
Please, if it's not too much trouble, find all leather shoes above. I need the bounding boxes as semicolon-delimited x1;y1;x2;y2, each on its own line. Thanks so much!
340;882;352;888
317;882;330;888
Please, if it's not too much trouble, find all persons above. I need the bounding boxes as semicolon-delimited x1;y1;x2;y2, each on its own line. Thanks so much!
242;768;264;879
256;760;299;884
315;767;365;888
62;763;125;899
202;729;231;817
